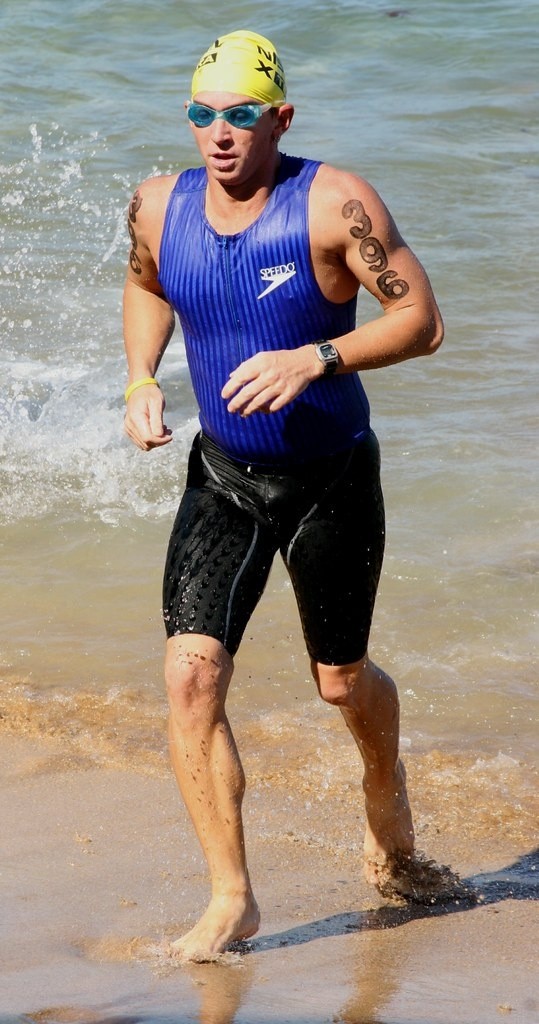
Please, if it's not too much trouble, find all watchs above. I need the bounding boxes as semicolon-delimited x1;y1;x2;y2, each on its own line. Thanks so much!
311;340;339;376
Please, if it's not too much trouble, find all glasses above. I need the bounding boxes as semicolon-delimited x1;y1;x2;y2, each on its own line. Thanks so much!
187;101;272;130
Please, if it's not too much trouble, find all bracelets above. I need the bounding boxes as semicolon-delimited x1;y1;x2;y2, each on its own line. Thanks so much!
124;378;159;402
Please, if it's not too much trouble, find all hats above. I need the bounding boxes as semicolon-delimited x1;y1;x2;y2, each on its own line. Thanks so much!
191;30;288;108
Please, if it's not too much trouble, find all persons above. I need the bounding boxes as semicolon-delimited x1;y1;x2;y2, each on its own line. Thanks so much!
121;27;446;954
